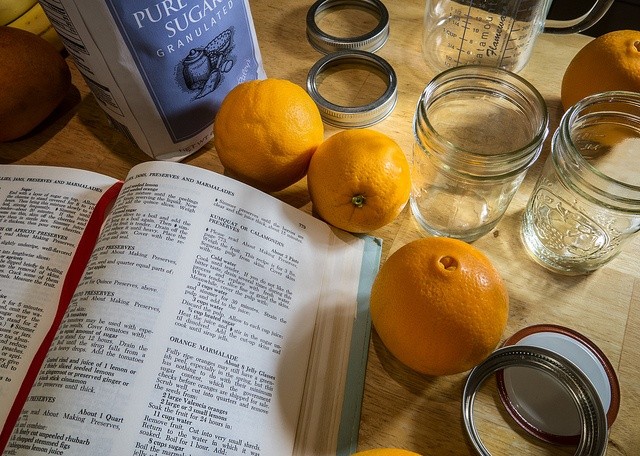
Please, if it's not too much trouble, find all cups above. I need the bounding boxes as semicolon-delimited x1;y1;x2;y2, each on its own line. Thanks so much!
420;1;615;80
412;64;551;254
523;85;636;277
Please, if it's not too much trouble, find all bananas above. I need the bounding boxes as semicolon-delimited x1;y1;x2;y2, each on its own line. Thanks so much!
0;0;63;35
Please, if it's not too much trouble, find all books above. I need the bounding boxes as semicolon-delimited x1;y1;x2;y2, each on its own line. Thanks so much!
1;156;385;456
